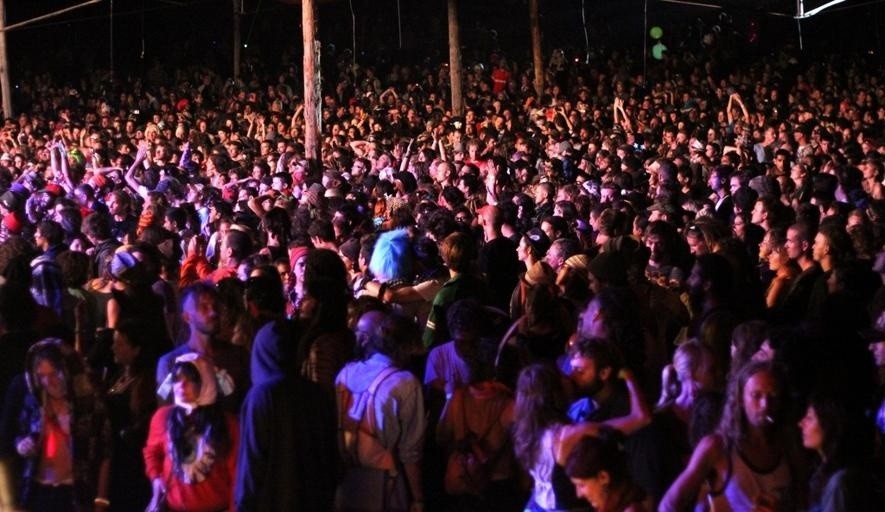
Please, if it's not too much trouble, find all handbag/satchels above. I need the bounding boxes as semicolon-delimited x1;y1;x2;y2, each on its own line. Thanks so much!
333;468;410;512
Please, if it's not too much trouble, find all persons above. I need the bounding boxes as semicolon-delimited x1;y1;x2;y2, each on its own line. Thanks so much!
2;1;885;511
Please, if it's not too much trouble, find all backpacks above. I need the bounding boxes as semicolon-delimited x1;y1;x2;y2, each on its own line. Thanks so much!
444;388;513;499
